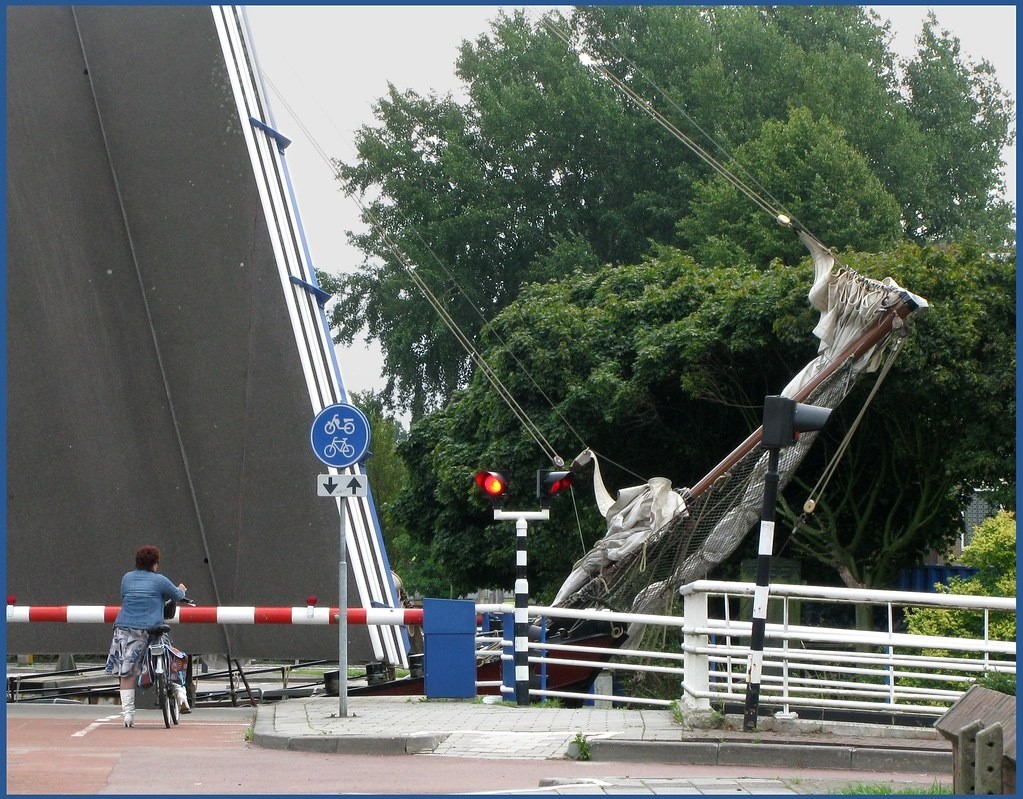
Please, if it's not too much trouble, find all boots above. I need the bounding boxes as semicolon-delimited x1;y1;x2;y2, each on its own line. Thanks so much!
120;689;135;727
172;683;191;714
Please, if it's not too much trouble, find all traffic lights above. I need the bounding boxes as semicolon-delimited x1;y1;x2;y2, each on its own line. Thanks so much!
476;470;506;499
762;397;834;449
542;472;575;496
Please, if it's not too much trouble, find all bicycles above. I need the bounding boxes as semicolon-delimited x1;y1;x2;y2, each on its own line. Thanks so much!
147;598;197;729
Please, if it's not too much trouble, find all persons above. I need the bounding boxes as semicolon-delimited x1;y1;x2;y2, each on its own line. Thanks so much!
103;546;192;729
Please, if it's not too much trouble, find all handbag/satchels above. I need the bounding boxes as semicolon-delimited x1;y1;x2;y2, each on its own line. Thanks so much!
161;644;188;685
138;643;158;689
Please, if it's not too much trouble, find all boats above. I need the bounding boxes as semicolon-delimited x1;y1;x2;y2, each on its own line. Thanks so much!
0;3;934;707
